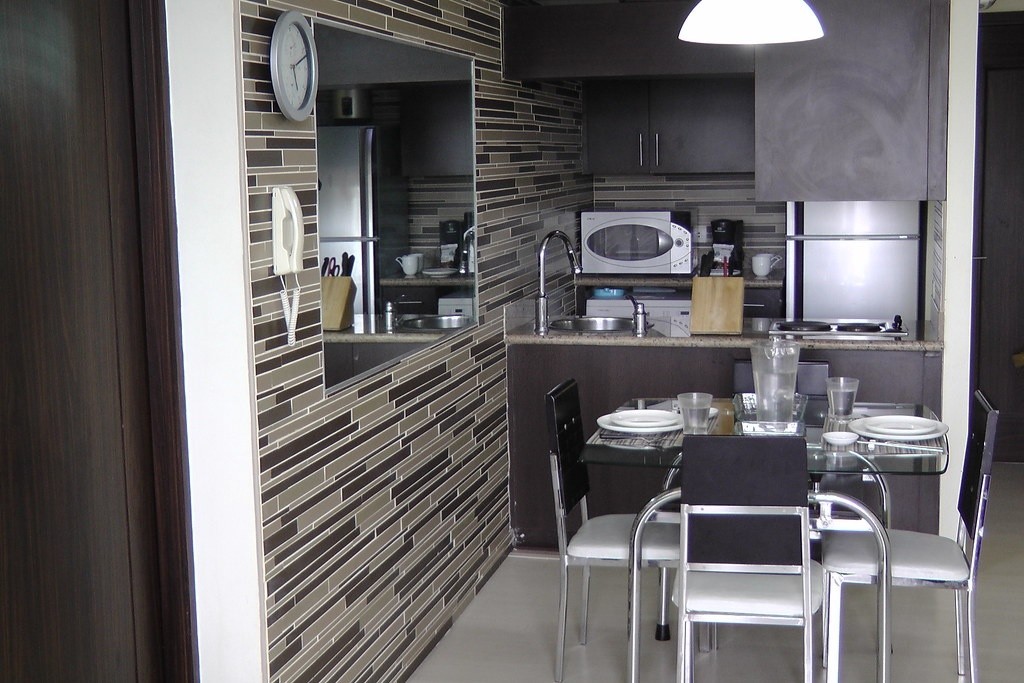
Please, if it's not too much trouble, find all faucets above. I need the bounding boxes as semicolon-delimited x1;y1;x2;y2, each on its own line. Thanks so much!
623;295;647;337
385;301;424;334
459;231;475;274
533;230;583;336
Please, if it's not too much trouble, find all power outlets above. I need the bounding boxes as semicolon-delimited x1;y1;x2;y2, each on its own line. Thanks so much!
692;225;707;243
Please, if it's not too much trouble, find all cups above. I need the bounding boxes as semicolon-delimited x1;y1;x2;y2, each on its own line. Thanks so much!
825;376;860;424
751;340;799;422
751;253;782;280
395;254;424;276
677;393;713;435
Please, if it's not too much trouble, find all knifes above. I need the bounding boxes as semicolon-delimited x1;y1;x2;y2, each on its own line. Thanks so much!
321;252;354;276
700;251;736;277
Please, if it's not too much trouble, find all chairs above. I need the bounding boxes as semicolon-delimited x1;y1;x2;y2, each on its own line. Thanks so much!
733;360;829;511
671;434;829;683
819;390;1000;682
547;379;718;682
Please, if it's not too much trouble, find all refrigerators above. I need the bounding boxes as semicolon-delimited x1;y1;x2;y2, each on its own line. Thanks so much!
803;201;920;321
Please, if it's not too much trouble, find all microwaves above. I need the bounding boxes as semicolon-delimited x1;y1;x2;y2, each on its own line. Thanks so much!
581;207;698;274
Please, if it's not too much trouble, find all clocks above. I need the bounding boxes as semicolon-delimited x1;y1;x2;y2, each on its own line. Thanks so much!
269;9;319;123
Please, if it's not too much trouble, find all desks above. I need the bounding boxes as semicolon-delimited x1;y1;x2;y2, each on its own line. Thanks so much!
577;397;949;683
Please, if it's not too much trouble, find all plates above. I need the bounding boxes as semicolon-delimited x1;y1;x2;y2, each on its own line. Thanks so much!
848;415;949;440
822;432;860;446
422;268;457;277
597;409;683;434
711;268;740;276
709;408;718;418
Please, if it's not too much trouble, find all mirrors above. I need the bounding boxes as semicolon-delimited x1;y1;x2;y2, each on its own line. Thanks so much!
311;16;480;391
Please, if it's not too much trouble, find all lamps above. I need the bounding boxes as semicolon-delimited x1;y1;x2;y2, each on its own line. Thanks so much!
677;0;824;44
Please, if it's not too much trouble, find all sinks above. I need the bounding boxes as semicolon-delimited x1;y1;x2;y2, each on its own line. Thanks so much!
548;316;655;335
399;316;475;332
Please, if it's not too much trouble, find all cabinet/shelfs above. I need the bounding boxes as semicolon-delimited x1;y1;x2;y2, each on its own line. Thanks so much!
585;77;755;175
382;286;438;315
754;0;947;201
401;83;474;176
744;288;784;317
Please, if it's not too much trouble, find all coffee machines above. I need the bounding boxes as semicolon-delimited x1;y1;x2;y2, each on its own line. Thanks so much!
437;220;466;276
710;219;744;275
